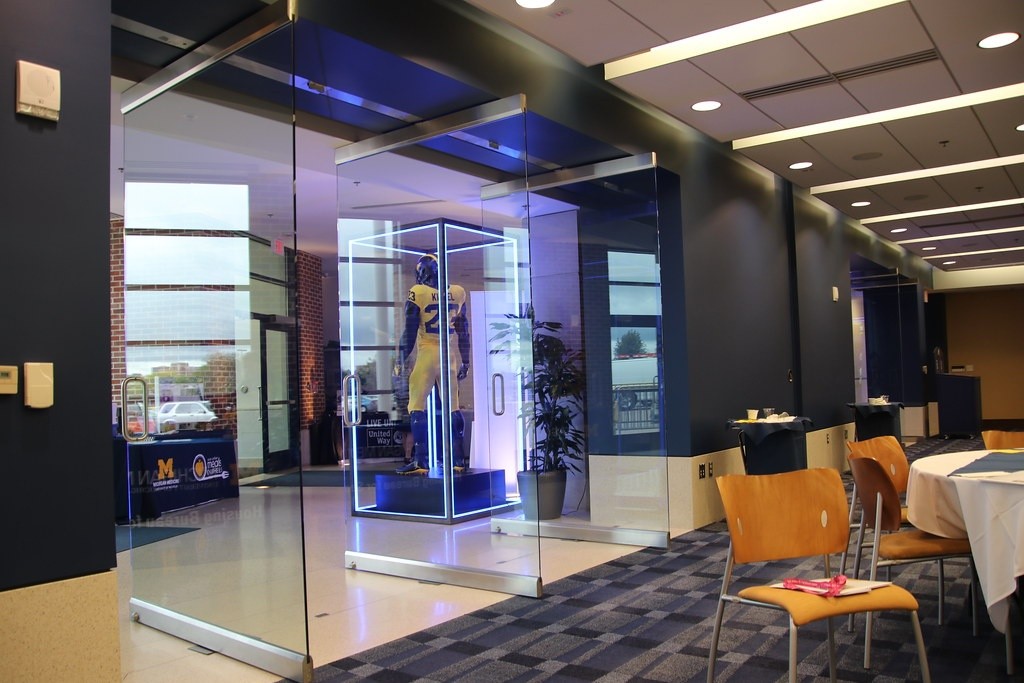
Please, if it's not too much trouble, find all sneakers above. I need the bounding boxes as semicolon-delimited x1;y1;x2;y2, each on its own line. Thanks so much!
453;462;466;473
395;461;430;475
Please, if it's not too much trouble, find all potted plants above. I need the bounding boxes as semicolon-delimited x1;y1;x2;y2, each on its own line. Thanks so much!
486;303;587;522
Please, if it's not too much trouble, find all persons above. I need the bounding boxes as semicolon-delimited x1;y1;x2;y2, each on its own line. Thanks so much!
394;255;470;476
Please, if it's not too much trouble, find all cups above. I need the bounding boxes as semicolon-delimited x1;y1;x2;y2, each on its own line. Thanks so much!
882;395;889;402
746;409;759;420
868;398;875;404
763;408;775;419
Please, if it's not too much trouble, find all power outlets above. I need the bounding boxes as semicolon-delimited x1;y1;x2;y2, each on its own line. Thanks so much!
827;434;829;443
699;463;705;478
709;462;713;476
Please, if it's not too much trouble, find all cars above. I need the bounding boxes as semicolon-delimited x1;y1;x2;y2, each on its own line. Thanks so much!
125;401;218;434
342;395;378;413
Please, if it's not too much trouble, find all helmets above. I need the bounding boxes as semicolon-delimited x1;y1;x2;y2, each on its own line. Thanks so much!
415;253;438;283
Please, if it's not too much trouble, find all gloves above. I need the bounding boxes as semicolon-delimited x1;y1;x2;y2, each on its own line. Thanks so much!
456;364;470;381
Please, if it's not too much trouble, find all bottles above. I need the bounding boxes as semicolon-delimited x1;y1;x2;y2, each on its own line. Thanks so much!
933;344;945;373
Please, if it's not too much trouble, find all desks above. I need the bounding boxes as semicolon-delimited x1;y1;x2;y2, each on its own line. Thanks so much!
846;401;907;443
726;418;813;475
905;448;1024;676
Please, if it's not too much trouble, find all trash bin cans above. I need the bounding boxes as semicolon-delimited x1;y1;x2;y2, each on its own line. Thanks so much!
726;416;811;475
847;402;902;445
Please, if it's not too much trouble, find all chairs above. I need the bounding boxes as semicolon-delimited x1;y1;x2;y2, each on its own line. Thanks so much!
840;436;977;668
705;466;932;683
981;431;1024;452
